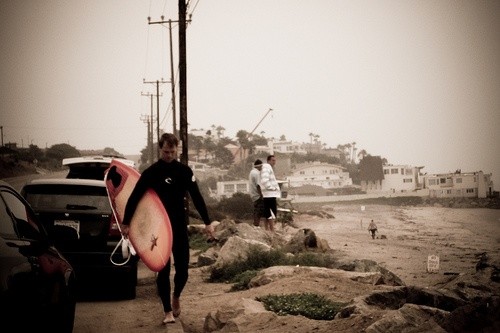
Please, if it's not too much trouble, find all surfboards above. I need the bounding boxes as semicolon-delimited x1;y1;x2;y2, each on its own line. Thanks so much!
104;160;173;272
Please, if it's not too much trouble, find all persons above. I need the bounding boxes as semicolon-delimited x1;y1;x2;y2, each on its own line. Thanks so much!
248;159;264;226
121;133;216;324
260;155;281;230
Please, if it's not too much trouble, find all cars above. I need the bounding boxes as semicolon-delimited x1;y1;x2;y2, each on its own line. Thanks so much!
0;180;77;333
62;155;135;181
19;178;141;300
